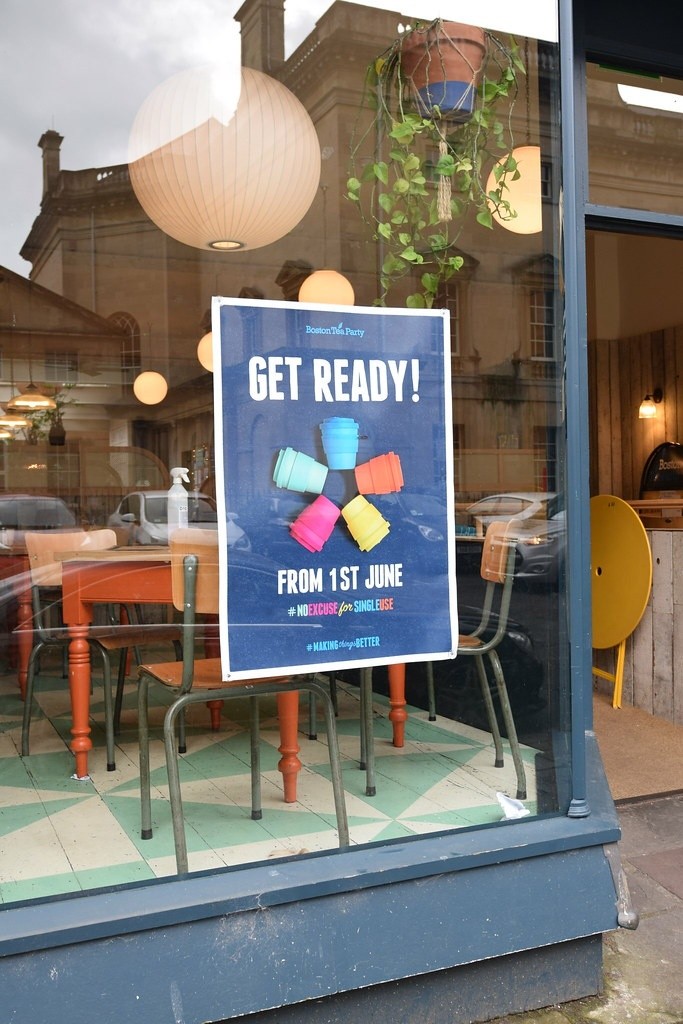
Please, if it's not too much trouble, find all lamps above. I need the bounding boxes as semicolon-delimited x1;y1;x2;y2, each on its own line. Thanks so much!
638;387;663;418
483;146;562;233
196;332;212;372
126;63;321;251
133;370;167;405
0;386;56;440
297;267;354;303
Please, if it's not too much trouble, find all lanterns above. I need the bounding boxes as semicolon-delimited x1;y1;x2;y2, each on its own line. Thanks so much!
485;146;542;234
297;270;355;306
197;332;213;373
127;63;321;253
133;371;168;404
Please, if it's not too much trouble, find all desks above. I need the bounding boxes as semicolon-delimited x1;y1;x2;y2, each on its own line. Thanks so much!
591;494;653;706
624;499;683;529
0;546;135;702
51;545;409;805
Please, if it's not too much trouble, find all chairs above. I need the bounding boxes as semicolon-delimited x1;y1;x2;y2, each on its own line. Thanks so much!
357;516;530;801
20;530;187;771
137;529;348;873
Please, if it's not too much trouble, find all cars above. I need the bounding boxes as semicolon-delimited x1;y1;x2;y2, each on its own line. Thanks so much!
106;489;252;554
233;487;567;586
0;495;92;554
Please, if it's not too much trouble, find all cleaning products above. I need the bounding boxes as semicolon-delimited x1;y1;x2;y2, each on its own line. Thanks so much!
167;467;190;548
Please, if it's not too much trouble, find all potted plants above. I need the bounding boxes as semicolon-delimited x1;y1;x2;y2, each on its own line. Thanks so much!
345;18;530;310
38;382;79;445
